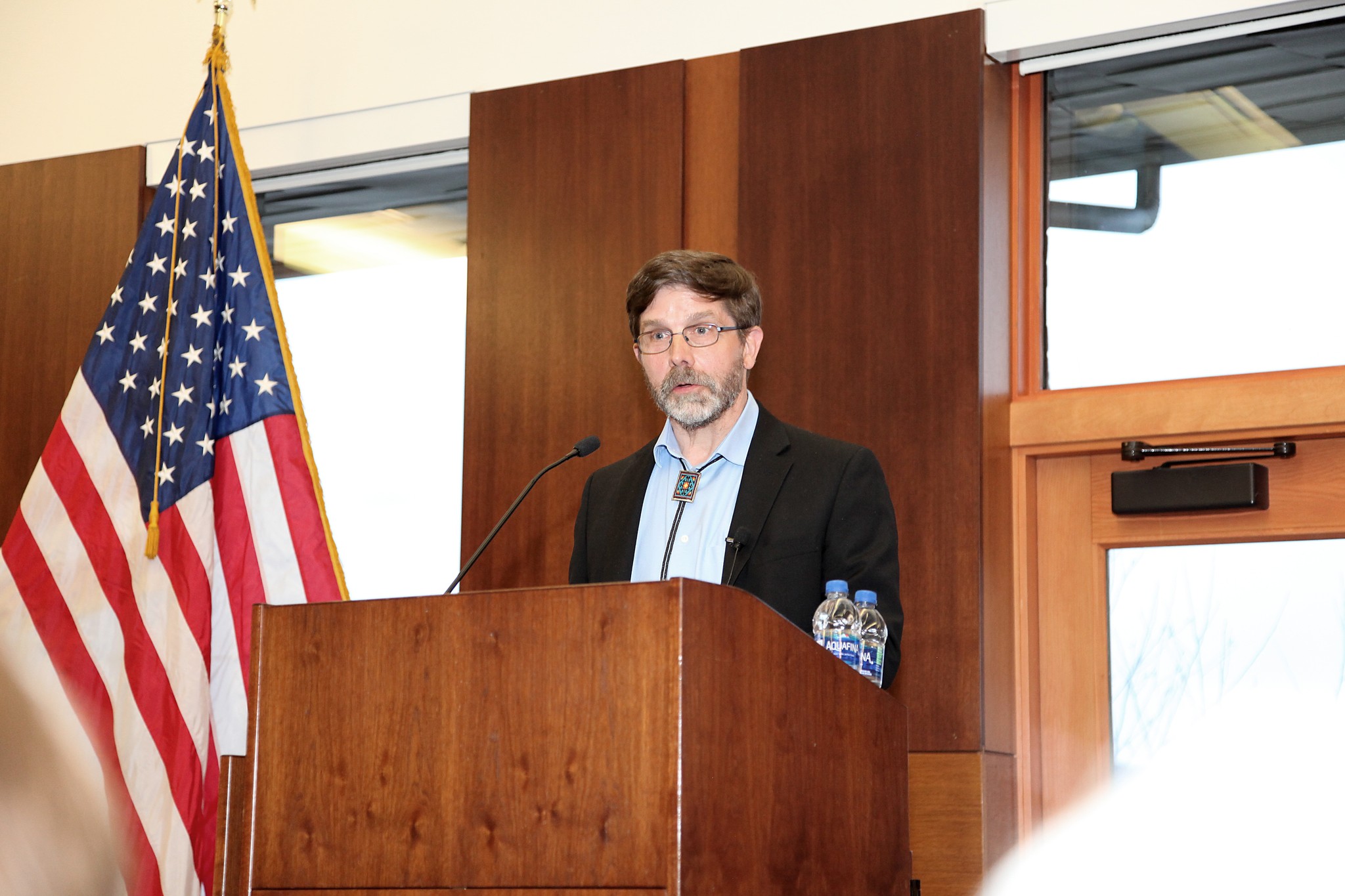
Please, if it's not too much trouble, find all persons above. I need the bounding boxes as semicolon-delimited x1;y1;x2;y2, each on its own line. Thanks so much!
569;249;904;694
0;673;119;896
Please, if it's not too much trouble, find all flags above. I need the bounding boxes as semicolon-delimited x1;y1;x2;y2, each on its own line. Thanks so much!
0;47;348;896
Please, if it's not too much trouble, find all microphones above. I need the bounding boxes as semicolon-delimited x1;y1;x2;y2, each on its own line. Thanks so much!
444;435;602;593
733;528;751;552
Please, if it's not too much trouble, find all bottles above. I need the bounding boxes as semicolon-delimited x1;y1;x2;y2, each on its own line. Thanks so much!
812;580;862;674
854;590;888;688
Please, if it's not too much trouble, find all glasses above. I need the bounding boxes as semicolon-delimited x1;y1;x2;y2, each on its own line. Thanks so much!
636;323;750;354
